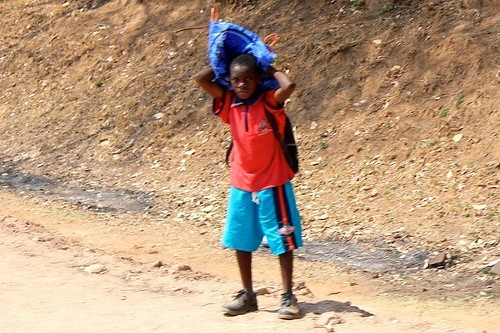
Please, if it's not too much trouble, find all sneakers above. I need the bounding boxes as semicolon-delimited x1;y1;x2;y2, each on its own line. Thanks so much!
223;289;257;315
278;293;300;319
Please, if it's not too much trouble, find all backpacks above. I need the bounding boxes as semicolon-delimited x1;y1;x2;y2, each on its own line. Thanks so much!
225;91;298;173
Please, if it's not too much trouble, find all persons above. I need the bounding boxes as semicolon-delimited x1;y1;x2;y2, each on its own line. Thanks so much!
194;53;304;321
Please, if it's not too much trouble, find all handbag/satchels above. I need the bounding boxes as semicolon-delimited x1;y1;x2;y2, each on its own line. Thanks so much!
208;7;281;90
